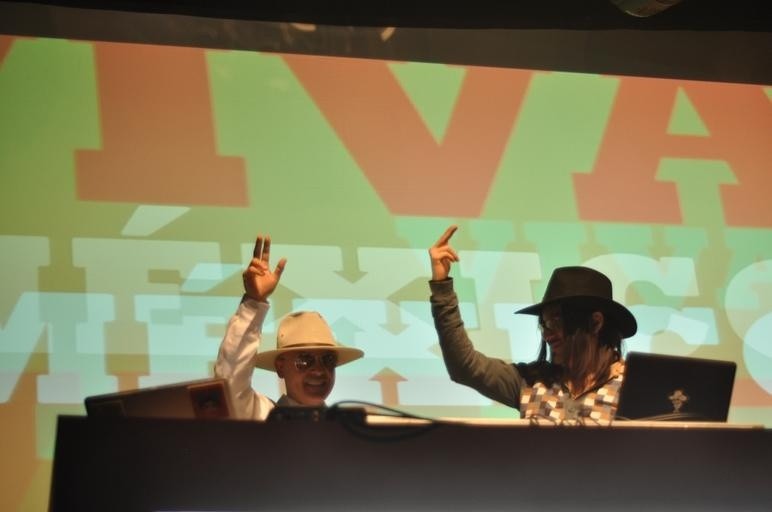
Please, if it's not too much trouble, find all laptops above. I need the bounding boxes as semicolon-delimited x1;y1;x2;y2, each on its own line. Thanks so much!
614;350;737;421
83;375;240;418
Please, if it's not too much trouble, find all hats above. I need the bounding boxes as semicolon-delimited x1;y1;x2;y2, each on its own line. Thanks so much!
255;310;364;371
513;266;637;338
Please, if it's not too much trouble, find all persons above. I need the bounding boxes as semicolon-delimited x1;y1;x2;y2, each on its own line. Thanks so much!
428;223;638;424
213;232;368;421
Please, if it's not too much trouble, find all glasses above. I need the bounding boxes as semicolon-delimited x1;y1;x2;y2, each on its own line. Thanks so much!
538;317;560;334
294;348;339;370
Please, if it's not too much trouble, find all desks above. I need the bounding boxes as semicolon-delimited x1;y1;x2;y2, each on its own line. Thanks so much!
48;412;771;512
